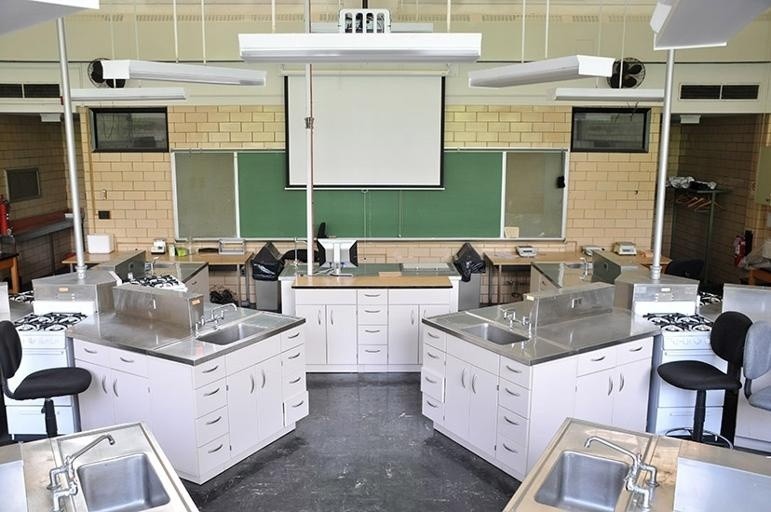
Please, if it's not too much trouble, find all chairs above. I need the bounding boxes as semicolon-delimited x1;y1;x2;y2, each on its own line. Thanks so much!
657;311;753;452
744;322;771;408
0;318;92;437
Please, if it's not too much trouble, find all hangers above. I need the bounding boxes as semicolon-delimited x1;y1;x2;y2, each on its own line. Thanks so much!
678;188;728;215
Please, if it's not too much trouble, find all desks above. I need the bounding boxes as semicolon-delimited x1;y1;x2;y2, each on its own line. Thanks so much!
62;251;254;308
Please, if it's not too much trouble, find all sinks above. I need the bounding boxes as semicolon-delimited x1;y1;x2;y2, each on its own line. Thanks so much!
159;268;195;283
77;452;171;512
534;449;629;512
565;263;583;269
287;265;318;276
461;323;530;345
195;323;267;346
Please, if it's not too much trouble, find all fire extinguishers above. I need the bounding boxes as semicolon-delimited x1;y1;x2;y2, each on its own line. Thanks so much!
0;195;13;237
733;231;746;268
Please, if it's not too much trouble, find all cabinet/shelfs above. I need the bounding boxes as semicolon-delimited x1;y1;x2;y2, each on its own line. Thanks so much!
387;290;449;372
72;339;148;431
445;333;499;460
575;337;655;433
494;354;575;481
357;288;388;373
227;333;285;458
279;323;309;424
295;288;357;373
419;323;445;430
148;356;232;486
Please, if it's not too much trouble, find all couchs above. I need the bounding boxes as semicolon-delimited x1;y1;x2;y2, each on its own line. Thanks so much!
0;193;20;294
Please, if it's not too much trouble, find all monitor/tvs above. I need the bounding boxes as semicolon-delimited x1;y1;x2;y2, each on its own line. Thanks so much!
317;238;358;276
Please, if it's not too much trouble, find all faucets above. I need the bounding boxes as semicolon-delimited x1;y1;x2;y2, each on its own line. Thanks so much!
46;432;115;510
580;257;592;279
584;435;657;511
150;256;159;275
497;303;529;326
293;234;307;275
195;303;238;330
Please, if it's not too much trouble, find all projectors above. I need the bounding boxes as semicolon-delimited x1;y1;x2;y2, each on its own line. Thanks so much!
338;8;391;33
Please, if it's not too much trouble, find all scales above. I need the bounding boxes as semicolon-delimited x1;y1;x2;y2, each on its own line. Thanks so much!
516;245;537;257
614;241;637;256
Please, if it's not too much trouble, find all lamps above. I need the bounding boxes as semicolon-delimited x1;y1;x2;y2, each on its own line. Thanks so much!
61;86;188;102
467;54;616;89
2;97;71;113
237;33;481;61
97;60;268;87
553;87;665;102
653;0;764;50
